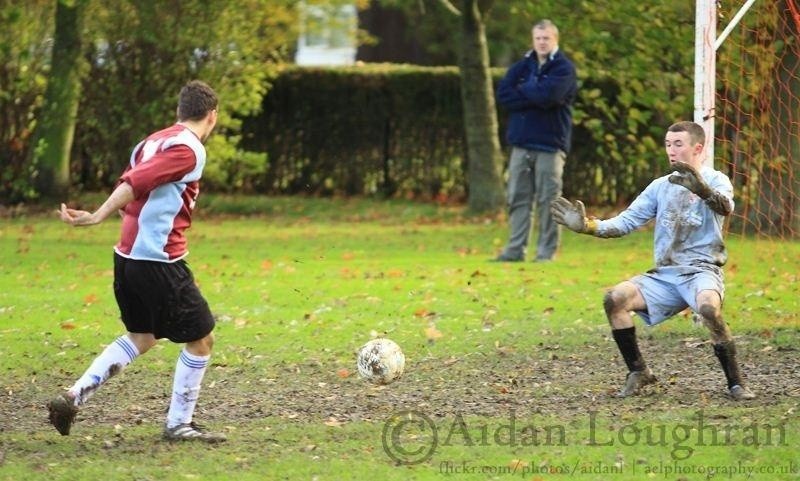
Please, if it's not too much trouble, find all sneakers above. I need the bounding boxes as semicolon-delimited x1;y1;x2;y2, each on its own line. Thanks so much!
488;254;555;264
46;392;82;436
728;384;759;401
614;368;660;397
162;421;227;444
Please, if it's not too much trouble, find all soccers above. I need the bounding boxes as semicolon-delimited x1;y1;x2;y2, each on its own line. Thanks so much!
358;339;405;384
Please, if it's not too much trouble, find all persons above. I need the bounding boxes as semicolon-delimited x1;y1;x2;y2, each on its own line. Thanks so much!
550;119;758;404
493;16;576;264
47;78;228;446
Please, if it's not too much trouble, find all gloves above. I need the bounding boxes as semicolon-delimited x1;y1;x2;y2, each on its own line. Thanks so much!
667;161;711;201
549;195;591;234
